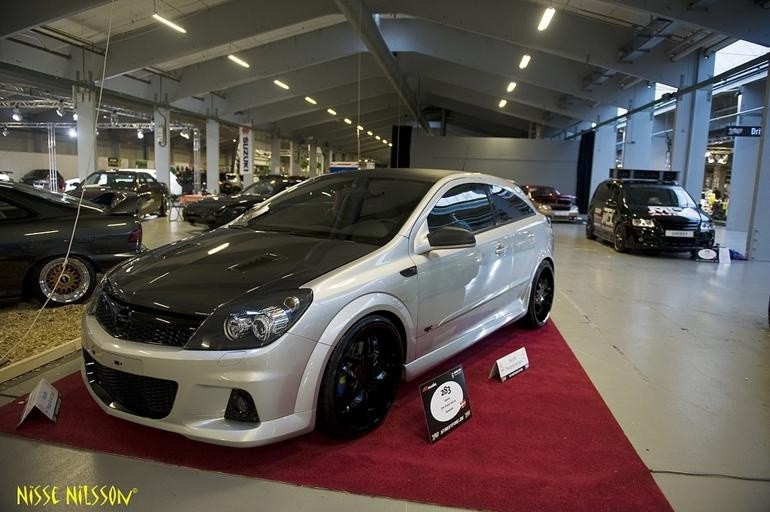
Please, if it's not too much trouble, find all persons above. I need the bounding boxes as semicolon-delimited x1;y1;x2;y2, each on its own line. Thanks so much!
713;187;721;200
723;184;729;202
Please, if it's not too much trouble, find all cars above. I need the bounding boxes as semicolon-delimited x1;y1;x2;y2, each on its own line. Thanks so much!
586;177;715;256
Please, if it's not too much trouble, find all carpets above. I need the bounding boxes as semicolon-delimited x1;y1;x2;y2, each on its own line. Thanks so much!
1;318;674;511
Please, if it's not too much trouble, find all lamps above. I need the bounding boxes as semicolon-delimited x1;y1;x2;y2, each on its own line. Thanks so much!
705;144;735;166
9;97;150;144
497;2;556;108
152;2;397;147
